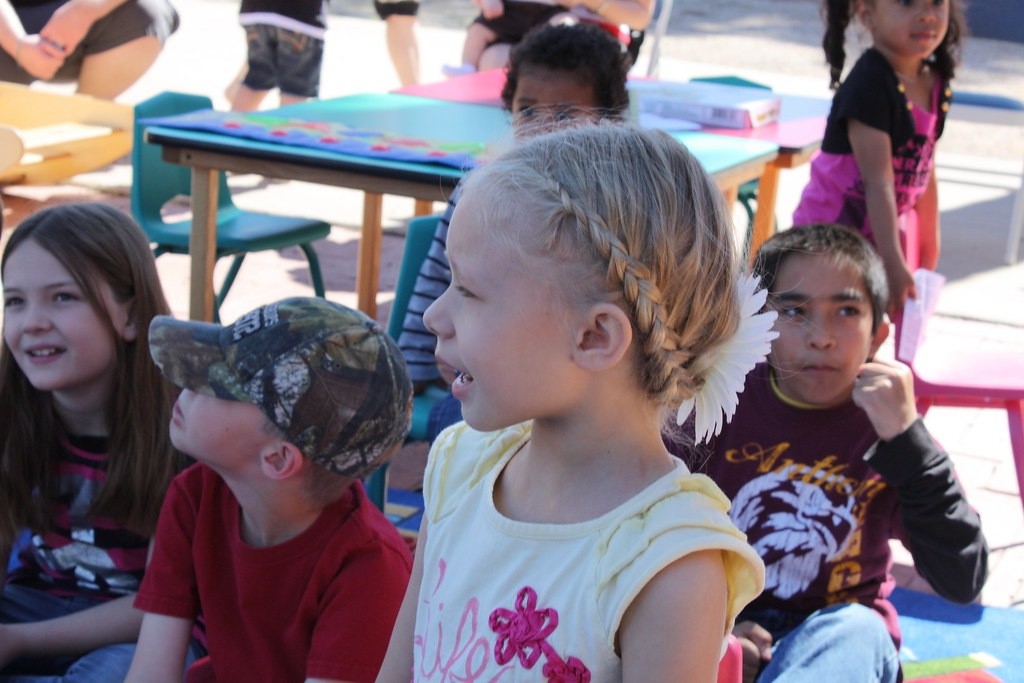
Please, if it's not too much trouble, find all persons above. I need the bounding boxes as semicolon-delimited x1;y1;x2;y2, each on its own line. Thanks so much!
793;2;966;319
370;118;768;680
231;0;327;113
440;0;652;67
661;215;985;683
396;10;632;485
0;202;213;682
121;296;419;680
0;0;176;95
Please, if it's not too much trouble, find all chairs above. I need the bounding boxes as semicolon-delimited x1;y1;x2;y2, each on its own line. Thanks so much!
867;212;1024;518
127;91;335;331
380;210;453;436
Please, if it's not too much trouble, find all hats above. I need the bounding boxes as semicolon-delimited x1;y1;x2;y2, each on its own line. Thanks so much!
148;295;413;487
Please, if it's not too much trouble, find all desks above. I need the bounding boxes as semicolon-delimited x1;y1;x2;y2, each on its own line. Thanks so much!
143;92;778;408
388;66;845;273
